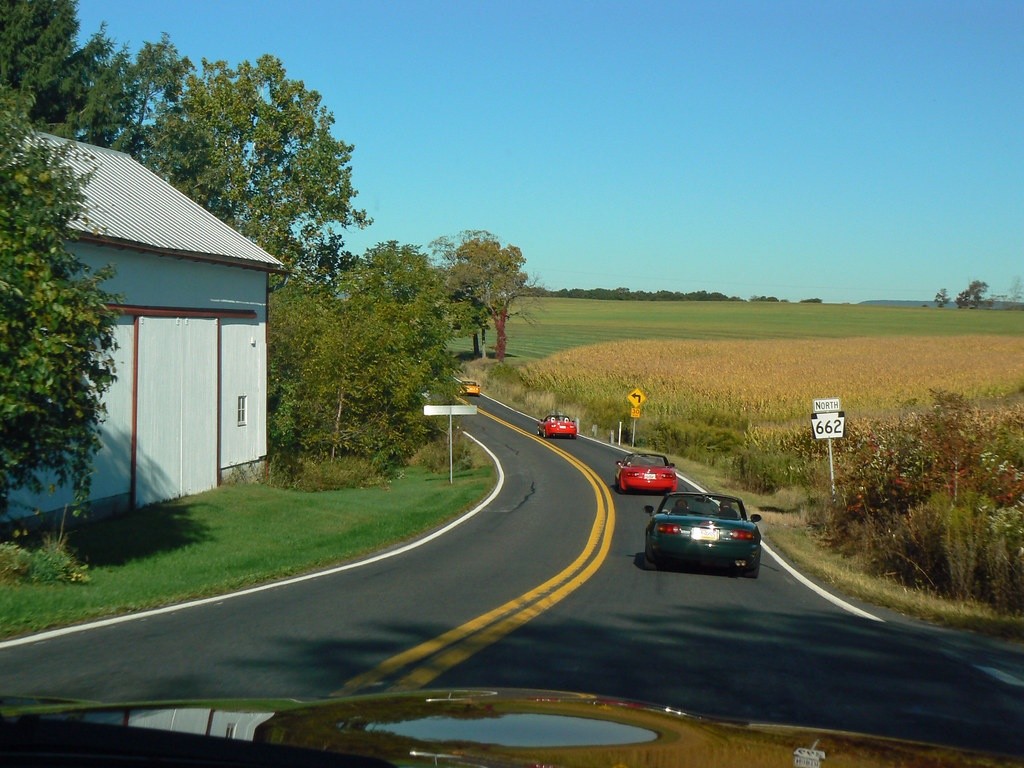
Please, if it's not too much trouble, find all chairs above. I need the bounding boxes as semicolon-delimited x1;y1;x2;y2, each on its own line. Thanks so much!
671;507;688;513
718;507;737;518
563;416;570;421
550;416;556;422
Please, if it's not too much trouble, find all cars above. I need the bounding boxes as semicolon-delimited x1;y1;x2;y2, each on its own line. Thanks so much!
616;453;678;494
537;414;577;439
460;380;481;397
643;492;762;578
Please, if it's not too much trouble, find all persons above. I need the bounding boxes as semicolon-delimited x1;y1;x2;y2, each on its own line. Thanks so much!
675;498;688;511
720;501;732;512
